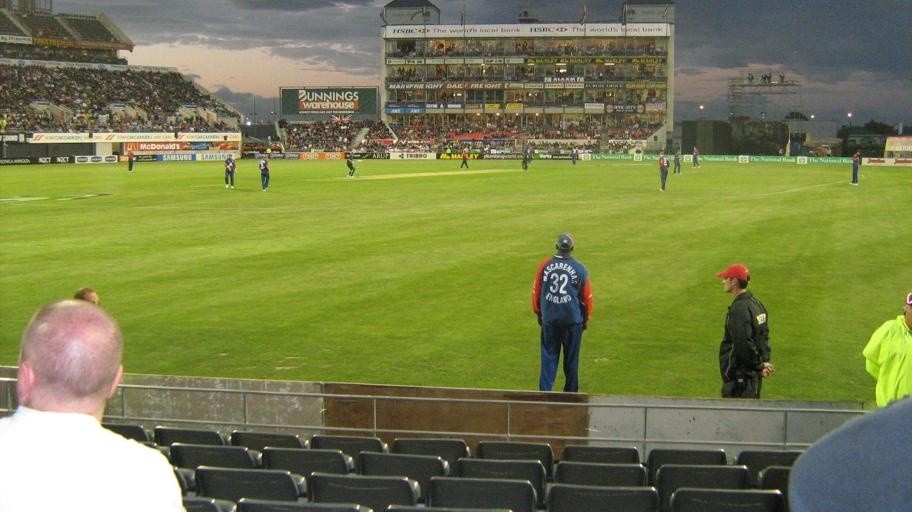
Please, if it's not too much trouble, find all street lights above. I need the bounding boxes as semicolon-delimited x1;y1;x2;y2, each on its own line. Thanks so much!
699;104;704;119
847;111;853;128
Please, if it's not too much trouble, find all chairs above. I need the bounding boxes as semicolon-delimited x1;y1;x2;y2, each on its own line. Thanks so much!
105;424;803;511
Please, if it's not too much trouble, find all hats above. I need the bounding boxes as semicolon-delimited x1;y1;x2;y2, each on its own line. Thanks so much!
556;235;573;249
717;264;750;281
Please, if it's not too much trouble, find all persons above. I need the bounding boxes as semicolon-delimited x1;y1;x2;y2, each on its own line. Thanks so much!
673;150;683;174
852;147;862;185
346;155;355;180
522;150;532;171
659;151;670;191
0;298;185;512
531;232;593;393
693;145;699;168
570;146;579;164
461;150;469;168
1;0;243;134
225;155;236;189
242;120;384;154
383;26;674;159
714;262;775;399
72;288;99;307
863;293;912;408
127;147;134;172
259;156;271;192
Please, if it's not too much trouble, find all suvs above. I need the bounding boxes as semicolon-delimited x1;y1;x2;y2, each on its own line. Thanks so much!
806;145;831;157
